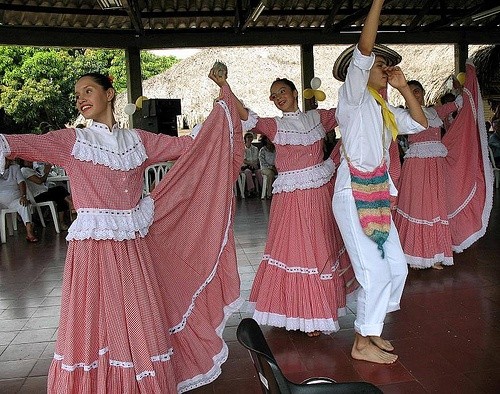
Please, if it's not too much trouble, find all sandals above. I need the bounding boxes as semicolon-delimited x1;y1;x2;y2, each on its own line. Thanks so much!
26;234;38;243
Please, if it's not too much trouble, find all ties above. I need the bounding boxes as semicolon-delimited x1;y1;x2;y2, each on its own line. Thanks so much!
367;87;398;142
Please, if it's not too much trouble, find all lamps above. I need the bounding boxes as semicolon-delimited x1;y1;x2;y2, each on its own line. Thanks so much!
252;0;267;22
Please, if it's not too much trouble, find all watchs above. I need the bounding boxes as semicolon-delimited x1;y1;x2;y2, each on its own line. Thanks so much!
20;194;26;198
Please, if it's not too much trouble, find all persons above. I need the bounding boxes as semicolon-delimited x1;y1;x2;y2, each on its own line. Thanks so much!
440;78;463;133
332;1;429;364
0;72;245;394
323;93;500;170
208;65;400;338
39;122;53;135
258;135;278;200
242;133;263;199
391;58;495;269
20;159;71;230
0;158;39;242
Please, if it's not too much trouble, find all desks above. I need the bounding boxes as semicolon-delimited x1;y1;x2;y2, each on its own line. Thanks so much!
46;176;70;194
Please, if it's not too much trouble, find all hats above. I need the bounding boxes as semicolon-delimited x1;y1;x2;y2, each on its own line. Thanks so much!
332;42;402;83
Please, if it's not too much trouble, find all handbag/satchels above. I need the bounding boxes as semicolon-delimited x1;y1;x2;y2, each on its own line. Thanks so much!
350;160;391;259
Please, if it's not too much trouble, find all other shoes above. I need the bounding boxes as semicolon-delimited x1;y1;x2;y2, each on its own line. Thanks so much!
58;224;68;230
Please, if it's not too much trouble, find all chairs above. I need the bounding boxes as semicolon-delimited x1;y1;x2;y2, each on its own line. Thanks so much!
0;146;500;243
237;318;383;394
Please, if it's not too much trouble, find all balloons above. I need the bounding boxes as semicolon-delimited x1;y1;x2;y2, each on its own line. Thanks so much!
123;104;136;115
457;72;467;85
314;90;326;101
303;88;314;99
311;77;321;90
135;96;149;108
